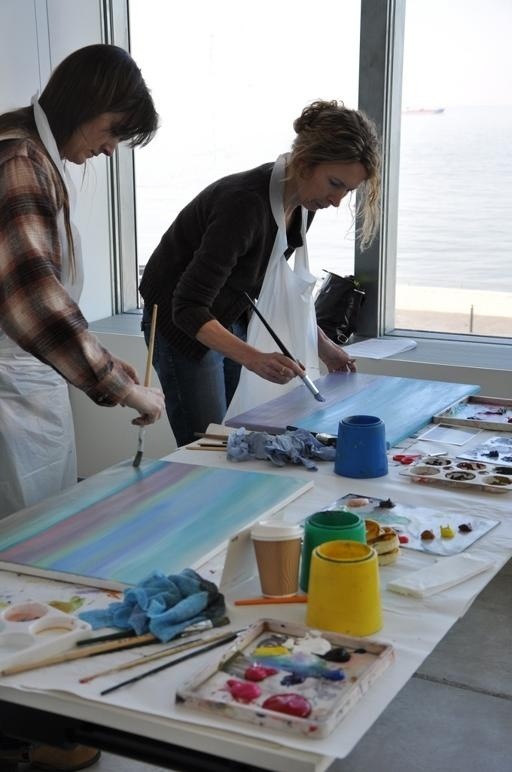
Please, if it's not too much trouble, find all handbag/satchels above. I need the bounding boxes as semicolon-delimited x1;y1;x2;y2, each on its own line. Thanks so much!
316;272;368;343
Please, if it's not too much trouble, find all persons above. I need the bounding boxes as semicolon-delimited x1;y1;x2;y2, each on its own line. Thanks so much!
0;42;169;521
135;97;387;449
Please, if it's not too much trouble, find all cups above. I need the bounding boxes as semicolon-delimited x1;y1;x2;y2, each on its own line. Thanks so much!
246;519;304;599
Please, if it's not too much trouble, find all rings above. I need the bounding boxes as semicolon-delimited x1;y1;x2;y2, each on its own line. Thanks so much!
279;366;287;376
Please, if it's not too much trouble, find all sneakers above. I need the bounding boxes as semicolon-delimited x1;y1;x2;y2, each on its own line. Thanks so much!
2;742;98;771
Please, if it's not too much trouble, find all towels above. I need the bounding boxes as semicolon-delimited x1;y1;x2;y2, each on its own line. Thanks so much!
93;566;227;644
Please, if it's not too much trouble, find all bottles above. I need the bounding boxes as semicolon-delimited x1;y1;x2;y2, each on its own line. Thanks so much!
299;509;369;592
334;413;389;478
305;539;385;637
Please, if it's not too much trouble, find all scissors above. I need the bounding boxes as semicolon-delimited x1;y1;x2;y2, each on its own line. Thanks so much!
393;451;449;464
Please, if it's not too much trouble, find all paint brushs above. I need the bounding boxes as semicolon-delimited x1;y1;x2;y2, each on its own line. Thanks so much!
133;304;158;467
241;291;328;402
0;618;248;696
186;432;228;450
285;426;337;445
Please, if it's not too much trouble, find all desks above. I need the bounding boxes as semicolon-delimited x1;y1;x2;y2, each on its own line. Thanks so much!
0;418;512;772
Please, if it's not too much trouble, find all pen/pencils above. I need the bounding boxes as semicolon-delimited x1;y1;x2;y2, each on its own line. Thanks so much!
236;596;308;605
